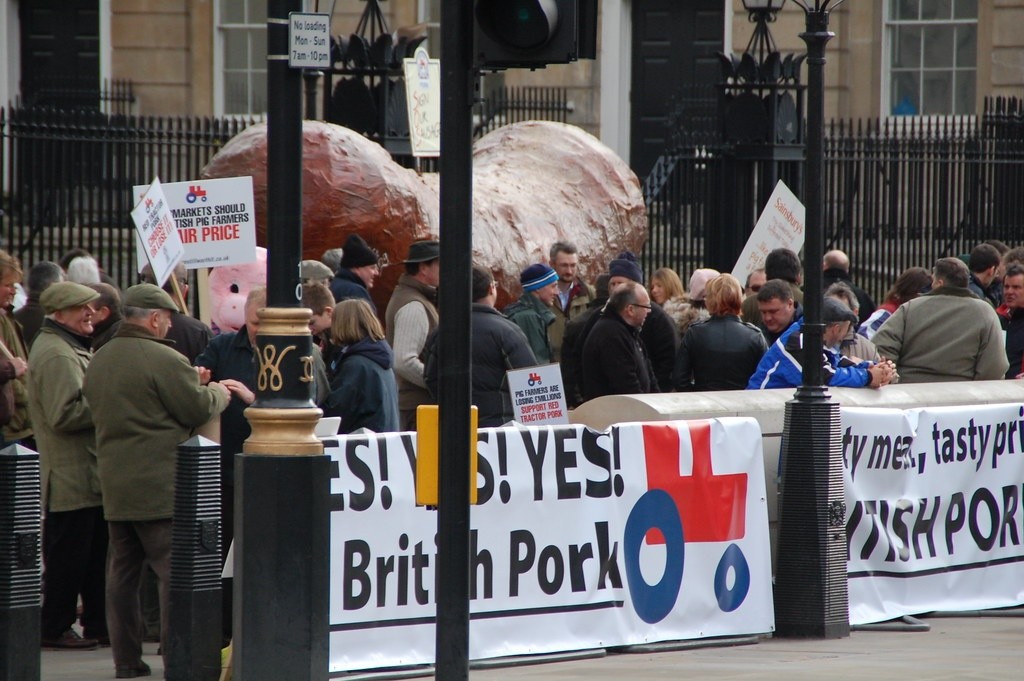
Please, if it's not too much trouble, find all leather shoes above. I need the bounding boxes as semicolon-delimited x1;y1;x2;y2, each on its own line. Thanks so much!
39;625;99;651
82;628;110;647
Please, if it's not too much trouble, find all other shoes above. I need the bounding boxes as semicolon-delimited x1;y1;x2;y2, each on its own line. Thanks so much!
115;664;150;679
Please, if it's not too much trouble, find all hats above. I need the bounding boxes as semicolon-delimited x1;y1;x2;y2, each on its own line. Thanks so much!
519;263;559;291
39;285;103;313
338;236;379;268
124;283;180;311
401;239;441;265
300;260;334;278
823;297;859;325
609;250;643;284
688;268;719;302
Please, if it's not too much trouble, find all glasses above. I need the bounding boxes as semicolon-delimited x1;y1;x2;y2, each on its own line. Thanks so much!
745;286;762;293
628;302;653;313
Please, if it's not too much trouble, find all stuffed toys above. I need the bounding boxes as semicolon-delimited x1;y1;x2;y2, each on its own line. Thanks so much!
208;247;267;333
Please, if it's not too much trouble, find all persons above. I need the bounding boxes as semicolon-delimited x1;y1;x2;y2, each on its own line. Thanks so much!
549;241;804;410
508;265;560;364
423;267;538;427
0;249;266;653
26;282;111;650
302;236;440;434
858;268;933;340
82;283;231;679
748;297;892;388
827;284;899;384
823;250;879;326
967;241;1024;380
871;258;1010;384
